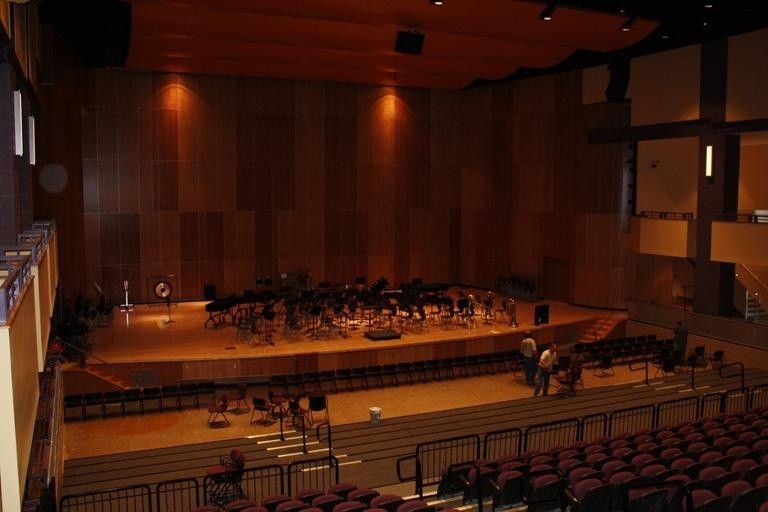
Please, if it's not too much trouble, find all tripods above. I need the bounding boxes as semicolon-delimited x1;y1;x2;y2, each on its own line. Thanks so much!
204;304;506;345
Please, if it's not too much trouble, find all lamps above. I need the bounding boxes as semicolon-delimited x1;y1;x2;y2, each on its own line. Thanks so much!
392;27;426;56
431;0;639;37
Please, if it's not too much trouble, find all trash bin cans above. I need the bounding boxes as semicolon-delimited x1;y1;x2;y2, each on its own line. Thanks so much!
369;407;382;424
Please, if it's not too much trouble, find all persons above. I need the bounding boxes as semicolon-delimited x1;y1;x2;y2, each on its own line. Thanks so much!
533;343;557;397
519;331;538;386
672;320;688;364
660;339;676;370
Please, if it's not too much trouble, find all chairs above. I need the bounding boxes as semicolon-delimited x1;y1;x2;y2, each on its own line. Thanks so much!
383;351;526;386
437;414;727;511
538;343;549;353
206;396;229;427
224;383;251;413
207;449;249;507
208;276;513;339
195;484;432;511
268;366;382;401
61;381;215;422
573;336;675;366
655;346;724;378
